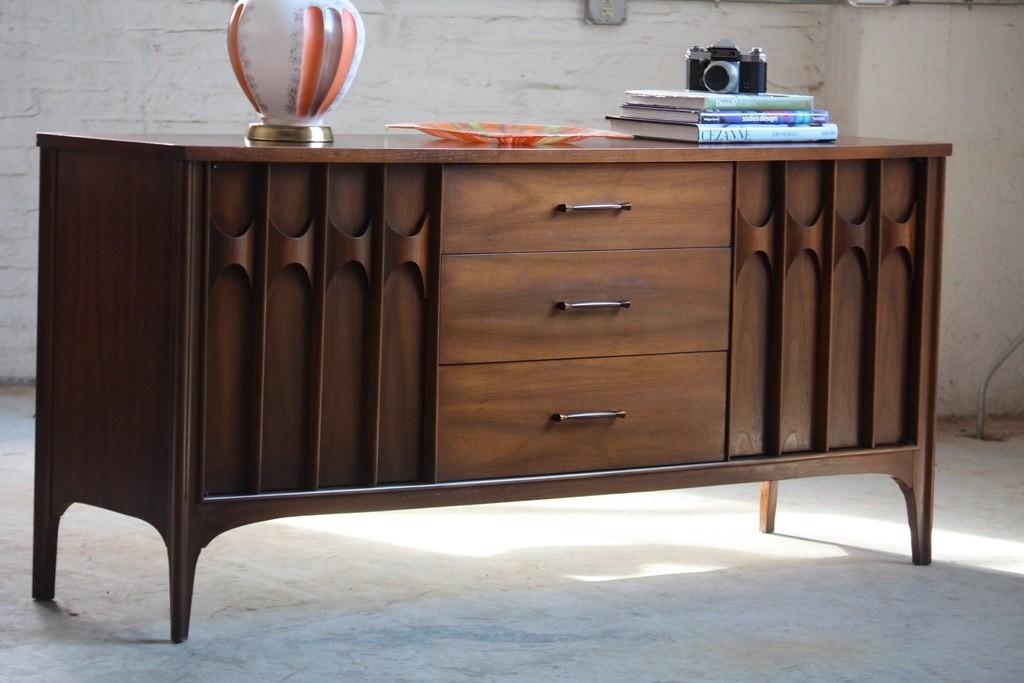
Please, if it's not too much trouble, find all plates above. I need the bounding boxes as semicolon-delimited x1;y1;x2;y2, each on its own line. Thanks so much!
385;121;634;147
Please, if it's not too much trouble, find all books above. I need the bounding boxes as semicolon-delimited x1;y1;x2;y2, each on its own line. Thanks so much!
606;123;838;144
621;93;829;125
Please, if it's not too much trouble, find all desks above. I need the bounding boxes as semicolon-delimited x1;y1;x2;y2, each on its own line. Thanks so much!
30;131;955;643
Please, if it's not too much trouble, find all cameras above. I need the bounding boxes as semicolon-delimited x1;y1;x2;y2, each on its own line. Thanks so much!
684;37;768;97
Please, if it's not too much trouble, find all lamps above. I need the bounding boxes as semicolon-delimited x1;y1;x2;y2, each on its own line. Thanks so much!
226;0;366;144
